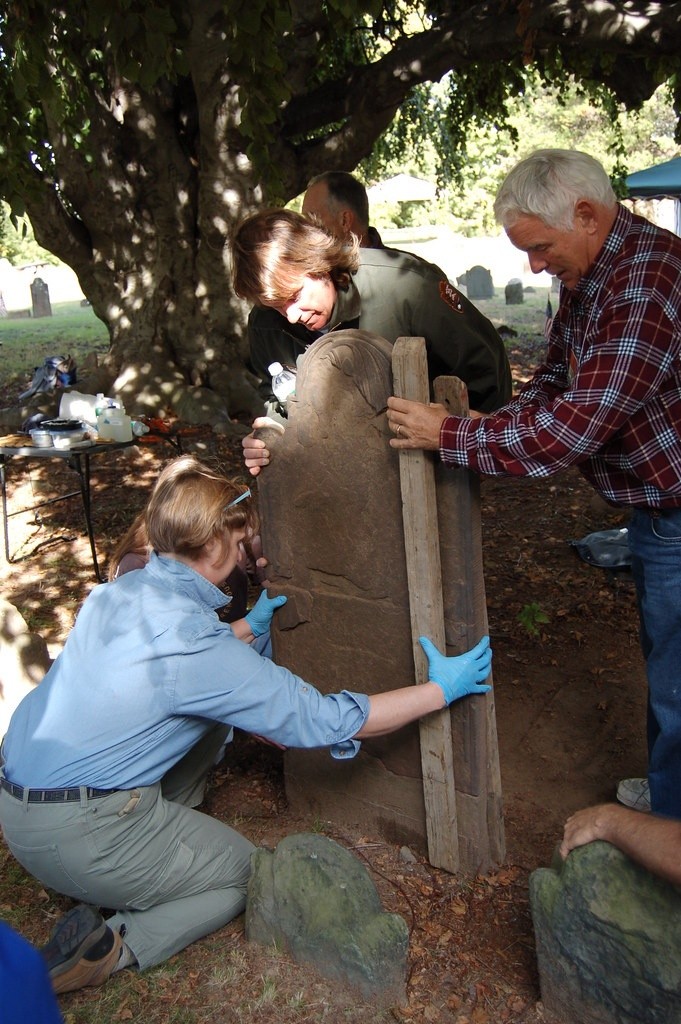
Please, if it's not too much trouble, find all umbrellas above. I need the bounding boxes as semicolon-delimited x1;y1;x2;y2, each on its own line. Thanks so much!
623;156;681;235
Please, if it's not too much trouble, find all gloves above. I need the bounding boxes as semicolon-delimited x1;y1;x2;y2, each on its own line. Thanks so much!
419;635;494;707
244;588;287;638
117;790;141;817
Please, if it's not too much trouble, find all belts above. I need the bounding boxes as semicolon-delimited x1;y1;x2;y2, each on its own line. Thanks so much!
0;776;119;803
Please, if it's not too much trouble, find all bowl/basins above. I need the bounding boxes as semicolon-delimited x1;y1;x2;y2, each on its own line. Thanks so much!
28;428;53;447
49;427;84;450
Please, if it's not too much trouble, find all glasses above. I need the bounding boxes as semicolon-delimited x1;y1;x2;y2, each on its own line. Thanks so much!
222;485;252;512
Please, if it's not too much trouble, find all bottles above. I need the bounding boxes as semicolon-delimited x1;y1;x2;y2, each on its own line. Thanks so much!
131;421;150;436
94;393;107;420
268;362;296;402
97;405;133;442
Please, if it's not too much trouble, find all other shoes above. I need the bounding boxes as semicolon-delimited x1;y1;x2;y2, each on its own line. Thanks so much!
38;904;123;996
616;778;652;811
576;528;634;567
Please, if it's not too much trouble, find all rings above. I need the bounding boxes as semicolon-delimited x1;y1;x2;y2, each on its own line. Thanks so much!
397;426;401;435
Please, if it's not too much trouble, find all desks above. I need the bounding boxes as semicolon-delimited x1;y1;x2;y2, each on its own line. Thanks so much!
0;431;183;583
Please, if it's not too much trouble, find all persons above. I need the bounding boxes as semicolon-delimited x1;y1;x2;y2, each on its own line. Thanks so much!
302;171;388;249
386;150;681;821
1;464;492;992
231;209;512;475
560;804;681;886
110;456;287;752
